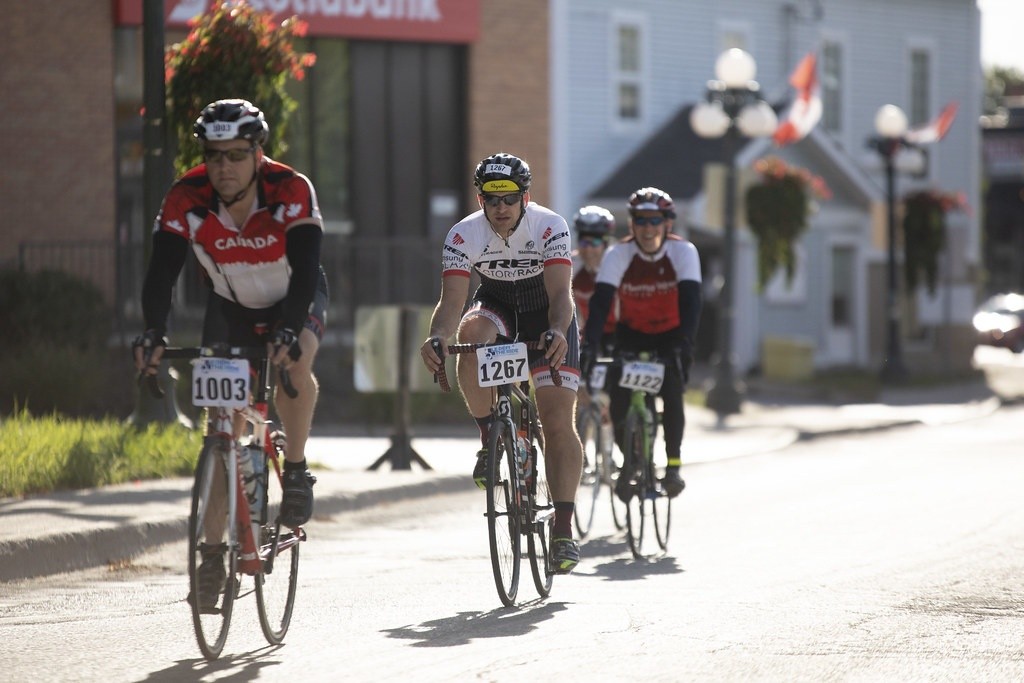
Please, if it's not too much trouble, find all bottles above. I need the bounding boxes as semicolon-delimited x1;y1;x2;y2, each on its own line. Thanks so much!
245;438;264;521
516;431;532;487
236;441;257;509
645;410;654;444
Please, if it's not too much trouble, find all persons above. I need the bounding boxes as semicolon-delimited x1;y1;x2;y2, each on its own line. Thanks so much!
420;153;702;571
133;97;330;612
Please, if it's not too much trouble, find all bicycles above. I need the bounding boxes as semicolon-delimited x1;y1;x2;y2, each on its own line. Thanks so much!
433;329;569;607
574;344;677;561
137;333;307;661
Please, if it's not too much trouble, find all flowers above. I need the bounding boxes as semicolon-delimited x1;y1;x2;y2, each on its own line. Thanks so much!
129;0;317;178
738;173;820;291
900;189;951;298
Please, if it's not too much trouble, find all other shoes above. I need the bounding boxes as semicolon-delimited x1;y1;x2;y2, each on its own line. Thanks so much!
616;460;641;502
661;466;685;499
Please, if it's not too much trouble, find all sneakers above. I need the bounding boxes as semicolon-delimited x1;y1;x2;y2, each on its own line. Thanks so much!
473;441;503;491
550;532;580;575
280;466;314;528
187;541;228;612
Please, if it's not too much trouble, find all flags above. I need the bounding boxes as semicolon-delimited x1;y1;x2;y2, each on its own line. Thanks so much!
773;55;823;143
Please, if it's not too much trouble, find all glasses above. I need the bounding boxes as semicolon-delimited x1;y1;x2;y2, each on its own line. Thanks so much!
631;213;668;226
201;147;256;164
577;239;602;249
480;191;522;206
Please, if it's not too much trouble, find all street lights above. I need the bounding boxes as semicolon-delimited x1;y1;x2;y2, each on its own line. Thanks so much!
688;46;778;414
862;101;930;402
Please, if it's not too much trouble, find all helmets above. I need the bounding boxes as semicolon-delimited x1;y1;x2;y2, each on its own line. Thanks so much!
626;187;675;214
192;98;269;147
573;206;617;235
473;154;531;197
